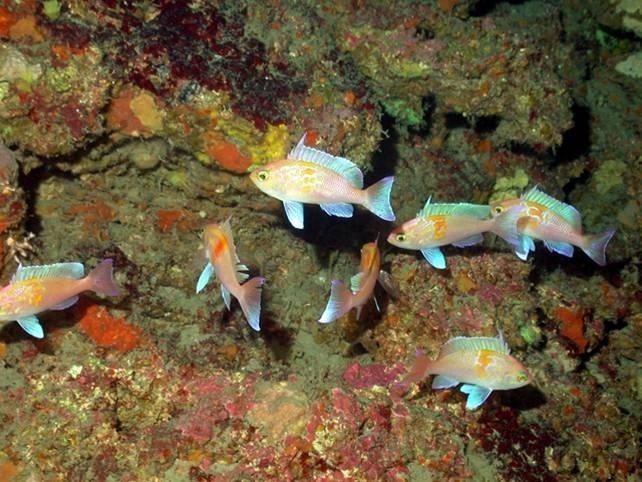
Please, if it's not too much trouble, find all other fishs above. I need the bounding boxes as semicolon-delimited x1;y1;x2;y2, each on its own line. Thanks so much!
0;257;119;339
